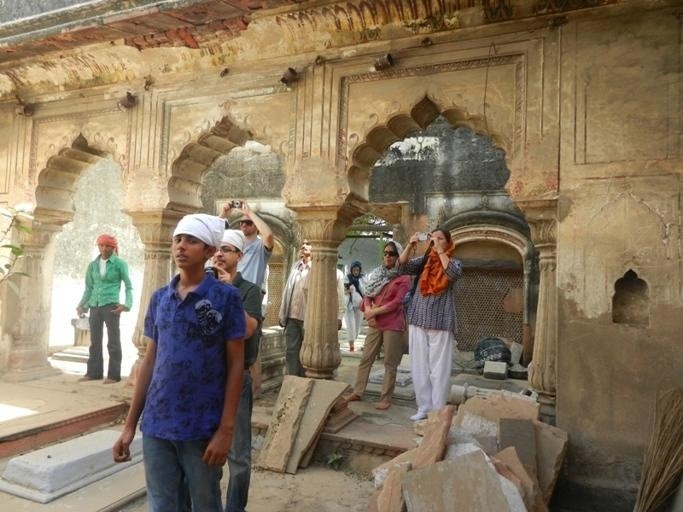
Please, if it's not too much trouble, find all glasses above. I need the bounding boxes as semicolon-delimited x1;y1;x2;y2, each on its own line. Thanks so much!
215;248;238;254
383;251;397;256
239;220;253;226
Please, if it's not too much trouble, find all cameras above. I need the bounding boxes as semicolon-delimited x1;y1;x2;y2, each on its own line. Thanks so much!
418;233;427;241
204;267;218;280
232;200;243;208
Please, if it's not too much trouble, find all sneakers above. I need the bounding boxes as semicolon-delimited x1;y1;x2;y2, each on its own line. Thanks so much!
345;392;362;402
409;412;426;421
376;400;390;410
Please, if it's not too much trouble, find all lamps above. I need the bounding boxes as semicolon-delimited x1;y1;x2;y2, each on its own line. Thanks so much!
116;91;136;112
374;53;394;70
13;94;34;116
280;66;297;84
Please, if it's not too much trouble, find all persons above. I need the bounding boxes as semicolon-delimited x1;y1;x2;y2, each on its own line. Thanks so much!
278;238;312;377
337;228;463;421
76;235;134;384
113;198;274;512
